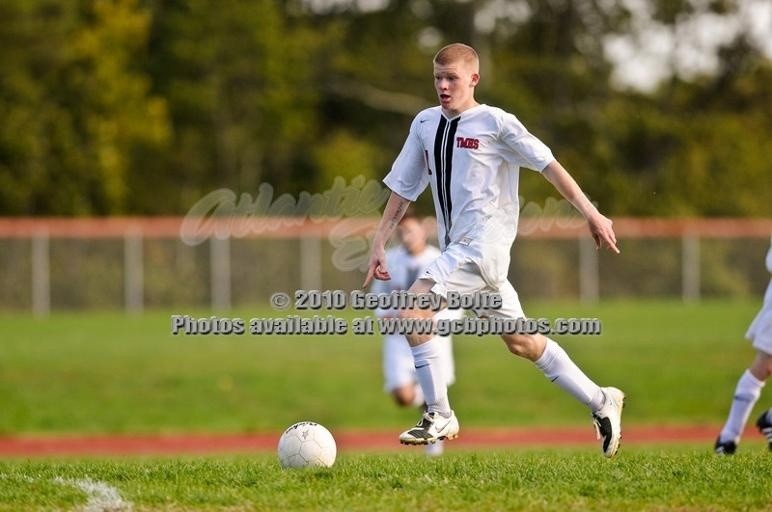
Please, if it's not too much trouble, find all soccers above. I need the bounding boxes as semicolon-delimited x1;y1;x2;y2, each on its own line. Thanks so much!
278;421;336;469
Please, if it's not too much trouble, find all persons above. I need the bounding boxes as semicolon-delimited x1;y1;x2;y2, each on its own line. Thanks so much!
366;213;457;459
714;246;772;456
362;44;625;460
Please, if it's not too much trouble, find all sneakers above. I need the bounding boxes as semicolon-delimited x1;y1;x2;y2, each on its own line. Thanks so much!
756;412;772;451
591;386;625;459
714;435;740;459
399;410;460;445
